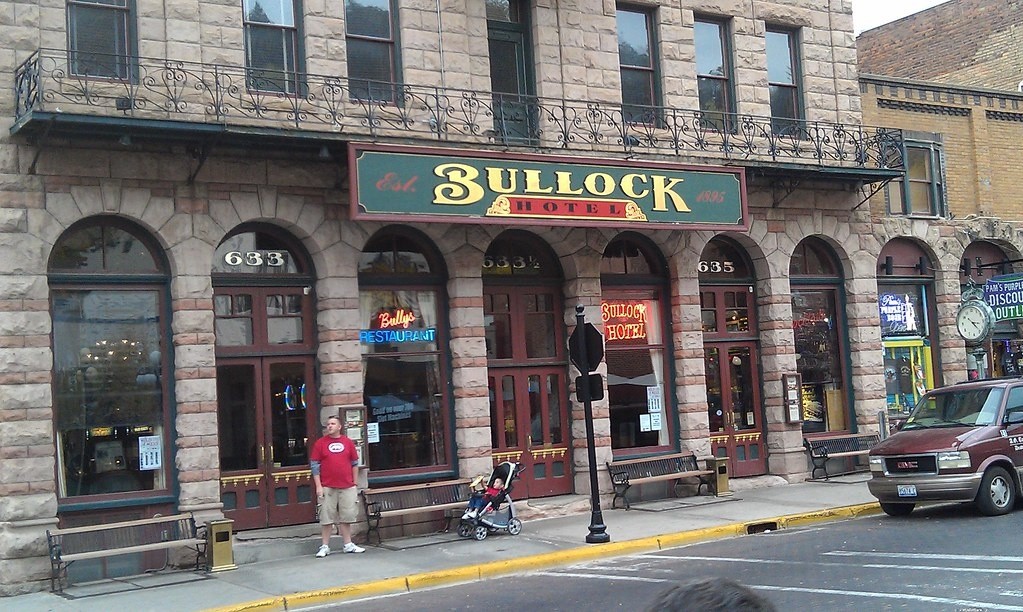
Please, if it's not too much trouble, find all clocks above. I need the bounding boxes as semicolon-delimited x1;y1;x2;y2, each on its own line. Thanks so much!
955;299;996;343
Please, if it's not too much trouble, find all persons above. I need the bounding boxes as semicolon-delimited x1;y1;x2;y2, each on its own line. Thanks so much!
310;415;366;557
462;478;504;519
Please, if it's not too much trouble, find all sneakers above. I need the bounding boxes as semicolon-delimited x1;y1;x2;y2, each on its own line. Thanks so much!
461;513;471;519
468;511;477;518
343;543;365;553
316;545;331;557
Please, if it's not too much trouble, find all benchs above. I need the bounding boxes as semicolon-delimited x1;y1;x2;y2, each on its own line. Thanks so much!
361;479;474;544
606;451;707;509
804;430;881;481
46;512;208;594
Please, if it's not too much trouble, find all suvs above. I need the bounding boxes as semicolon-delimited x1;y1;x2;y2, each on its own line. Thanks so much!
867;376;1023;517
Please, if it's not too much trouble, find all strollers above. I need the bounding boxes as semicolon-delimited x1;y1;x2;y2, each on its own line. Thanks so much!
455;461;523;541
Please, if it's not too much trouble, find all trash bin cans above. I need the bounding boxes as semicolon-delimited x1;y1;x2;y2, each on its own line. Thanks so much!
203;518;239;573
703;457;734;497
